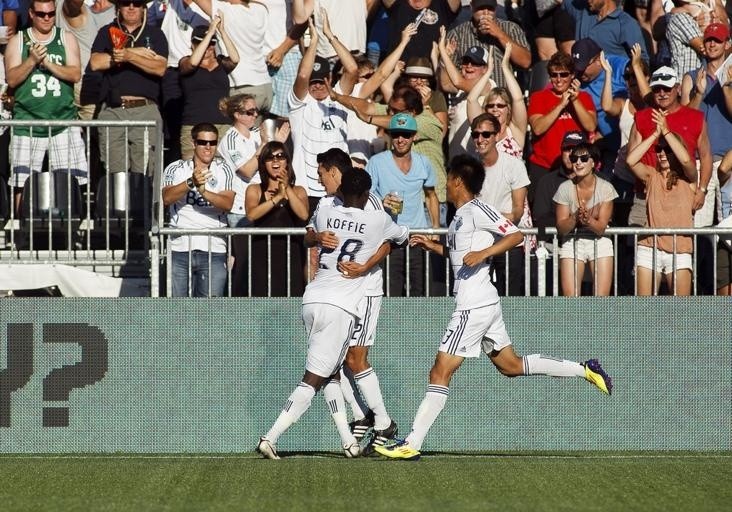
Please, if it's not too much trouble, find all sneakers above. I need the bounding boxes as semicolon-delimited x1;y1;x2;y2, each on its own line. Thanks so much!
581;359;613;396
373;438;421;461
349;418;374;443
343;444;363;458
362;420;398;457
255;437;281;460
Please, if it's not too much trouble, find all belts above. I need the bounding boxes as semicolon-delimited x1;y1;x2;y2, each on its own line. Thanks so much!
120;95;154;109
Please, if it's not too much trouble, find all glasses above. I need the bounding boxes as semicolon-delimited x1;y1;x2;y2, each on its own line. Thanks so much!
550;72;680;163
359;72;376;79
471;132;498;139
391;132;414;139
462;60;483;68
35;11;57;22
121;1;141;7
195;106;287;163
409;76;430;81
486;103;509;109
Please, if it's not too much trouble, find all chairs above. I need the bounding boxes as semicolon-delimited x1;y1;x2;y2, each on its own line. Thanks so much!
0;178;10;220
20;172;84;219
96;172;152;218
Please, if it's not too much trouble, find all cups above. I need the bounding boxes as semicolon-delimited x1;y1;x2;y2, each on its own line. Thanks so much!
387;187;406;215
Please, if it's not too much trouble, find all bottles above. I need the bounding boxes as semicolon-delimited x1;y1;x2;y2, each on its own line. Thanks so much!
144;36;153;51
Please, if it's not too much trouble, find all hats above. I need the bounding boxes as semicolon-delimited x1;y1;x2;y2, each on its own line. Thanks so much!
461;42;490;65
383;112;417;136
108;0;155;5
190;24;219;47
308;55;330;86
473;1;497;9
648;66;680;88
703;22;730;44
404;58;435;77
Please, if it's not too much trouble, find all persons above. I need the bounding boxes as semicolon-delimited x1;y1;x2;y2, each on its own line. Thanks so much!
303;147;398;458
374;153;613;461
2;1;732;298
256;167;410;461
